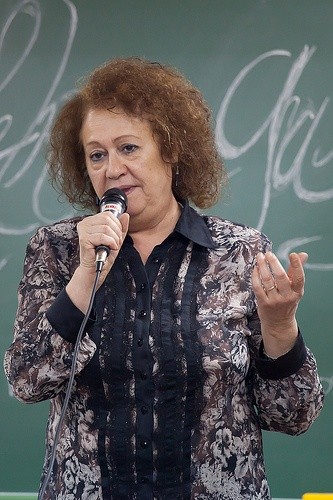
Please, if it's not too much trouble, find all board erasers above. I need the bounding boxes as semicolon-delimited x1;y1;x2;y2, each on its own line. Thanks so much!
302;492;333;500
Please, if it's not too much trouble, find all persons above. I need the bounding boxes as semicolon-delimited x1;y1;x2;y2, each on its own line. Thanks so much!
4;57;325;500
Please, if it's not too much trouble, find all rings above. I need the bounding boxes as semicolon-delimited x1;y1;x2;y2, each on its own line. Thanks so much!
265;285;276;292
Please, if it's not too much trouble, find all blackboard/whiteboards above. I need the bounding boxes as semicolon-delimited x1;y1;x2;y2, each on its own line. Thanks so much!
0;1;333;499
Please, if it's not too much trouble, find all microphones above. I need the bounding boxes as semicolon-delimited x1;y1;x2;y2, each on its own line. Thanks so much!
90;187;129;275
